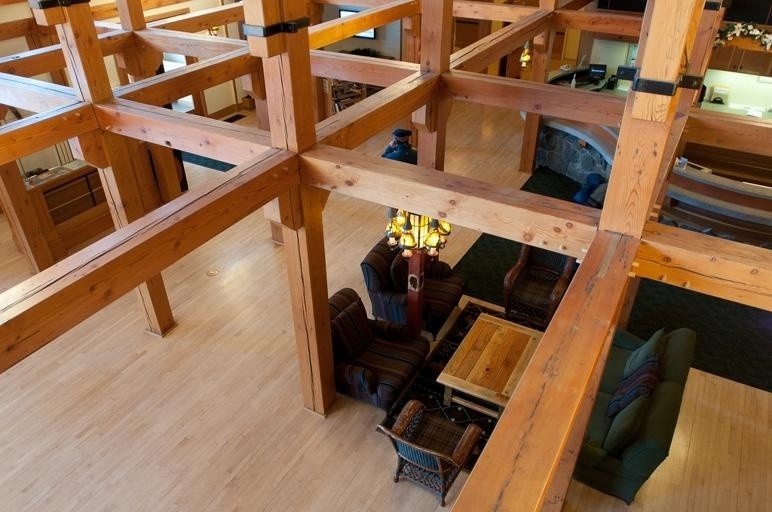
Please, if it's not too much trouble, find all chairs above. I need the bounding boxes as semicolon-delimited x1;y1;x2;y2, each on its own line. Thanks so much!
375;398;485;507
360;236;465;333
503;243;576;327
328;288;431;411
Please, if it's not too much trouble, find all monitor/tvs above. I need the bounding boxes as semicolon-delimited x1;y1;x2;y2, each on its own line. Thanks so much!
338;9;375;40
589;64;607;81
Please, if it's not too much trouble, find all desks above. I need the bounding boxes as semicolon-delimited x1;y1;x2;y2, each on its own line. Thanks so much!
436;312;545;424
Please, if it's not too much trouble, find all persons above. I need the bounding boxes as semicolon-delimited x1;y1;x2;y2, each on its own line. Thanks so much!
380;128;417;220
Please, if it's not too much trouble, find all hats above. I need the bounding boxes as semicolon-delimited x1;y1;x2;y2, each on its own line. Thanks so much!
393;128;412;142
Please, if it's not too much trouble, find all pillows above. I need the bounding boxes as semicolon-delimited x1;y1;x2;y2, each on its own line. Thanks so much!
331;301;373;349
602;395;651;455
390;251;408;292
622;328;666;379
608;354;660;417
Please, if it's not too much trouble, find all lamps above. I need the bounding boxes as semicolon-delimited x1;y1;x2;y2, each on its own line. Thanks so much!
518;40;532;69
386;208;452;259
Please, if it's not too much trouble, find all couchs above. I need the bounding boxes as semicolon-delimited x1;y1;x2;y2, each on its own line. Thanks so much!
571;327;698;507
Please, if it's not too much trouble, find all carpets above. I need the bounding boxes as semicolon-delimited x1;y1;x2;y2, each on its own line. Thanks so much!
377;294;548;474
451;164;771;390
180;151;235;172
